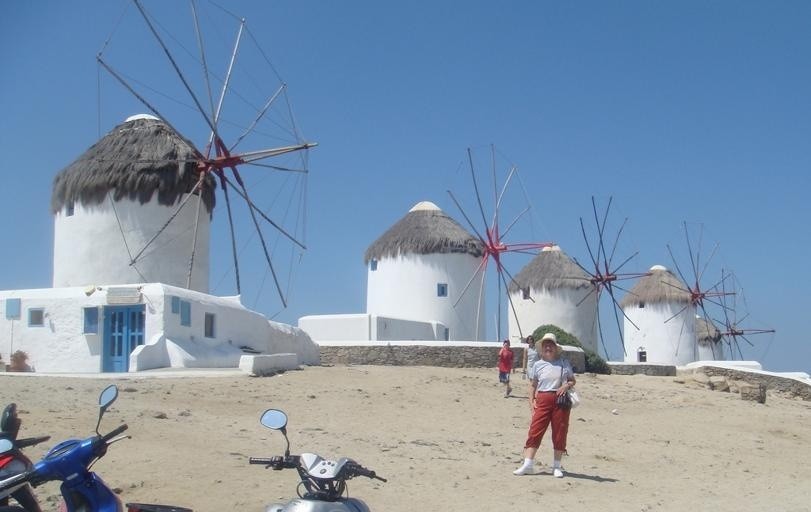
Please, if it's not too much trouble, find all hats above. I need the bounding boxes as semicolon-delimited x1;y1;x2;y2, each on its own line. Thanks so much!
534;333;563;356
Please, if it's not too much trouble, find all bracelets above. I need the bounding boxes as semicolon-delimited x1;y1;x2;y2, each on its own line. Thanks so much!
564;382;570;390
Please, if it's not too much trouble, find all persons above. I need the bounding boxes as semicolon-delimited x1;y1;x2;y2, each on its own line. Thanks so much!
498;340;515;398
522;335;540;401
513;340;576;478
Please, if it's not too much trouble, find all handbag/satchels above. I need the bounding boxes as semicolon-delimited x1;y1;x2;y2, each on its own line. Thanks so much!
556;358;582;409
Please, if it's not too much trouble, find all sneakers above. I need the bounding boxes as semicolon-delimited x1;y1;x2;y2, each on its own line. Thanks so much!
552;465;565;478
513;463;535;476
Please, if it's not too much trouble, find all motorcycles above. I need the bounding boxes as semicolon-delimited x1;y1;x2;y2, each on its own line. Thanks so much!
247;407;388;512
0;385;133;512
0;400;52;512
